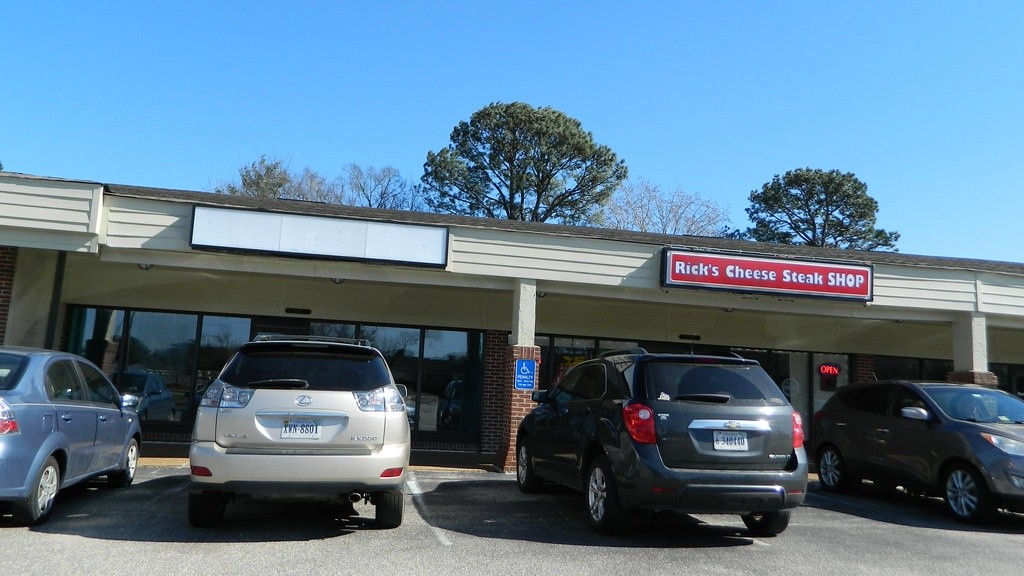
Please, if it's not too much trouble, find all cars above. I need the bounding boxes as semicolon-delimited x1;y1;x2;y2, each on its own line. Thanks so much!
96;370;177;424
807;380;1024;526
0;345;143;527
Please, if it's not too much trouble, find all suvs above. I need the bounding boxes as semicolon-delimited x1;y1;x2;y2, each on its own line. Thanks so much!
436;372;480;434
188;335;411;528
515;348;809;536
